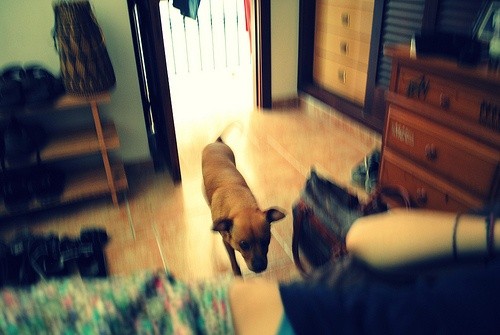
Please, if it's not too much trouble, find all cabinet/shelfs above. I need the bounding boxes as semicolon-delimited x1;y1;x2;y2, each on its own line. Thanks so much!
380;38;499;222
2;73;129;220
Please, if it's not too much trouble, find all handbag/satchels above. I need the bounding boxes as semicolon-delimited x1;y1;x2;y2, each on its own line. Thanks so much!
293;170;411;278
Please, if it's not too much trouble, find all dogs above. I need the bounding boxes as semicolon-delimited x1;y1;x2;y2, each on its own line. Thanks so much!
201;137;286;276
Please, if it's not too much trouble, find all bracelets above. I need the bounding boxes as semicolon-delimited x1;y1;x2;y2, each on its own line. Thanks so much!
482;208;497;255
449;209;466;260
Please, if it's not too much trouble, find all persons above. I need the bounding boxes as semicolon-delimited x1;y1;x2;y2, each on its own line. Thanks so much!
274;205;500;335
